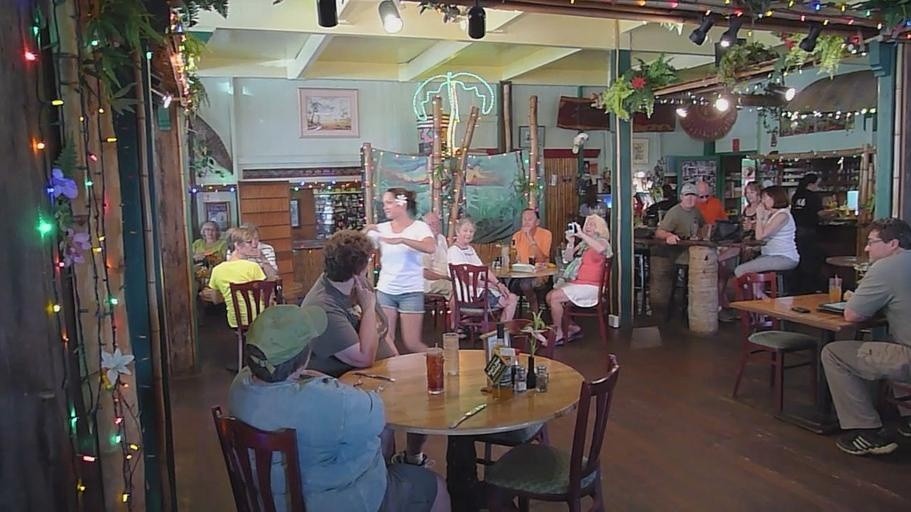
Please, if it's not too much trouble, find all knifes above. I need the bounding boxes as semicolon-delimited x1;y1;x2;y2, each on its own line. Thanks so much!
446;403;486;432
350;371;396;386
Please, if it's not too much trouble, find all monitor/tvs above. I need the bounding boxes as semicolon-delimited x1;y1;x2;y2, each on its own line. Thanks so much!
596;193;611;209
847;190;859;215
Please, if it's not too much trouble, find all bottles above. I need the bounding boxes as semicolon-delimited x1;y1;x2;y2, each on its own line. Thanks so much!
513;365;526;394
534;365;550;393
494;256;504;268
488;321;514;400
509;239;519;266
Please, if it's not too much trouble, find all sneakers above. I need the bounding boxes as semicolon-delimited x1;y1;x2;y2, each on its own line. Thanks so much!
836;428;898;455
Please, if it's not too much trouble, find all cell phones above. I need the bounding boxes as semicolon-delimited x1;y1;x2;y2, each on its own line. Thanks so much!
793;307;810;313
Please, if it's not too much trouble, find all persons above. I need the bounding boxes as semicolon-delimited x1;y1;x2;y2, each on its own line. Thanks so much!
416;210;519;324
192;220;282;328
541;215;614;346
653;172;830;321
228;302;456;511
508;208;552;322
356;187;438;356
302;227;399;378
822;217;911;456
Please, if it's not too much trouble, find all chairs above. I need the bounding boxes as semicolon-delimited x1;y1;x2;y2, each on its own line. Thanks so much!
449;263;500;338
210;404;304;511
483;353;620;510
424;294;447;330
632;220;782;325
474;318;557;475
562;255;612;345
229;277;283;374
735;272;818;414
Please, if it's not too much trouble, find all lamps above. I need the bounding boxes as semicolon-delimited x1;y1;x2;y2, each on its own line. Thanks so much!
316;0;486;39
688;12;822;55
674;73;795;117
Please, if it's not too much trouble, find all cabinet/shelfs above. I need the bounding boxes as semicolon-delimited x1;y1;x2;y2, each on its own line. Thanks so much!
752;146;874;215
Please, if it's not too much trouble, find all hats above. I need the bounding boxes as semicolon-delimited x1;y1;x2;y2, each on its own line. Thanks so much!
246;304;329;374
682;183;698;196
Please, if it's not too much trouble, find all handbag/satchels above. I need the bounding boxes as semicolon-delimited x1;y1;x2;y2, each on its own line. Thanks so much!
711;219;744;242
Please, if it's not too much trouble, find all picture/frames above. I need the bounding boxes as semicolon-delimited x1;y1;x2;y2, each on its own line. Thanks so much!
720;149;758;220
518;126;545;147
204;201;231;231
290;198;301;228
297;88;360;137
631;137;649;163
666;154;719;202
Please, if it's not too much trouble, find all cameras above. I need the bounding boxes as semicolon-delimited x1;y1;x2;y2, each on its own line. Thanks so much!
567;223;575;234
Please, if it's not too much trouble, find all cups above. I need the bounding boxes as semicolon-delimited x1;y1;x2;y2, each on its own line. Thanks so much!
529;256;536;266
424;347;444;397
828;277;842;303
689;218;699;239
441;332;460;377
700;223;712;242
